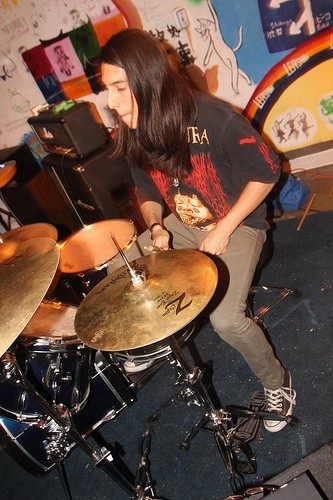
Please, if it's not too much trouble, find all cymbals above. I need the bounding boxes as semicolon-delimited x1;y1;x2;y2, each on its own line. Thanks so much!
0;223;58;241
0;240;61;358
74;249;219;352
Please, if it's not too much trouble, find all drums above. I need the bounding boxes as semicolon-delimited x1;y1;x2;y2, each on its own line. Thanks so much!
111;317;197;362
59;218;144;305
0;339;137;471
0;301;98;425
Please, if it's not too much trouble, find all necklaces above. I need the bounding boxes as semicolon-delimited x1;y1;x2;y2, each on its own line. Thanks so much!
168;172;183;188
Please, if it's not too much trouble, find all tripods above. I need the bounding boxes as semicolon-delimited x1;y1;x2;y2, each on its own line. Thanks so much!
166;336;298;495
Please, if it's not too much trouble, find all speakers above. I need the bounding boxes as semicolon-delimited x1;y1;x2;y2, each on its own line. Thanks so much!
238;440;333;500
41;141;149;237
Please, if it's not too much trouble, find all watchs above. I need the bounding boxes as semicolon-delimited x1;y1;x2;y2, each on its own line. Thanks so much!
148;223;161;240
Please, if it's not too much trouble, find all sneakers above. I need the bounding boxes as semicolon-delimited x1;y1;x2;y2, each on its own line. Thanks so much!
264;370;297;432
123;359;154;373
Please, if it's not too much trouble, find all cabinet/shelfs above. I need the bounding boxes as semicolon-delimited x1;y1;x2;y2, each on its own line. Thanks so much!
40;141;139;225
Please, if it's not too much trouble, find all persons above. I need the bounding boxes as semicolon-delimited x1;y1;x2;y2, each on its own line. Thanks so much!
107;29;297;433
